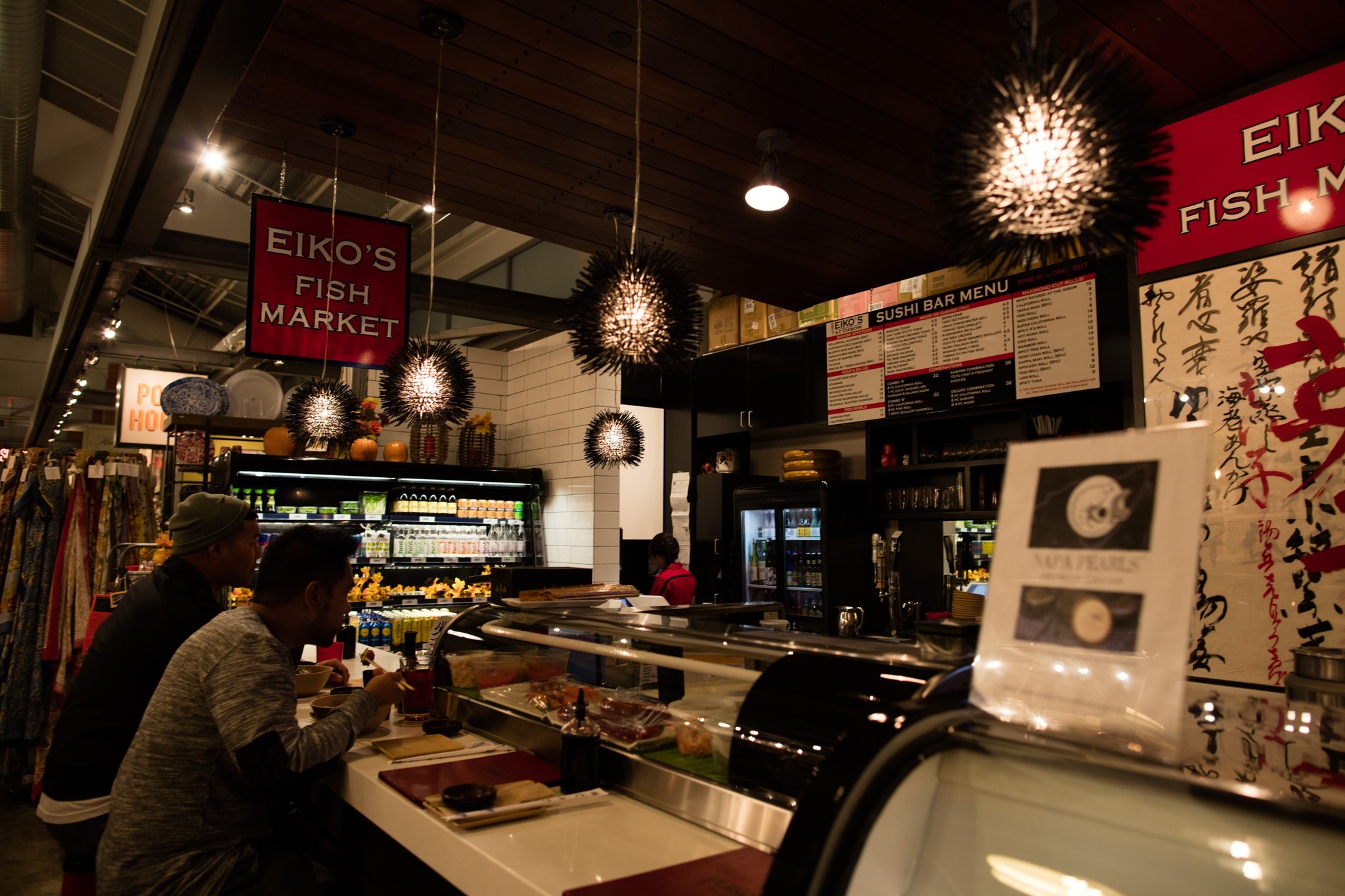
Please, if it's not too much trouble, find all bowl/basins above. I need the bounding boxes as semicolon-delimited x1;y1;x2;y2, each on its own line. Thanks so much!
522;649;570;679
310;694;350;719
668;684;751;775
760;620;788;632
493;646;539;678
294;666;334;696
447;649;493;688
952;591;985;620
472;656;519;691
277;501;359;513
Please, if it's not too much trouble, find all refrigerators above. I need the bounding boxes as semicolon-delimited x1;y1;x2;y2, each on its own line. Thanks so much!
733;477;865;631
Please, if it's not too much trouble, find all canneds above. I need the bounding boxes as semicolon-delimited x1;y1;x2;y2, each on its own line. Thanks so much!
456;498;524;520
527;502;538;519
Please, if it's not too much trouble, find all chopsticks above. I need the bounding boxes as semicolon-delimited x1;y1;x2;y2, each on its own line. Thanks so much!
359;654;414;691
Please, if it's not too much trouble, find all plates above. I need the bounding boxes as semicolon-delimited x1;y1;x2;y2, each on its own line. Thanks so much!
547;703;676;749
160;369;306;420
478;682;551;719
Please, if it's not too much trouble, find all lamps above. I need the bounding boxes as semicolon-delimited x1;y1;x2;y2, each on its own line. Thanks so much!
284;112;365;450
945;0;1170;284
742;129;793;216
173;189;198;220
378;3;476;432
582;390;648;473
559;0;707;380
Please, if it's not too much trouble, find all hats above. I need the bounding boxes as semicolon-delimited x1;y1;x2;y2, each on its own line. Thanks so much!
166;491;250;555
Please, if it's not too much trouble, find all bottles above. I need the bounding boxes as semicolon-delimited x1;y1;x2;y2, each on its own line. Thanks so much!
348;611;392;646
559;691;601;792
390;484;526;557
748;509;822;619
233;488;276;514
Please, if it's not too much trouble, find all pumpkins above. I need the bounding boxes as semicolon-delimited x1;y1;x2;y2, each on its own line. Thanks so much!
350;437;378;461
383;439;409;462
263;423;296;455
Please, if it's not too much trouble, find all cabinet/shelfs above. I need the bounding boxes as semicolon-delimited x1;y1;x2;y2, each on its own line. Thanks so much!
694;470;780;603
877;403;1040;520
694;323;865;445
154;415;282;528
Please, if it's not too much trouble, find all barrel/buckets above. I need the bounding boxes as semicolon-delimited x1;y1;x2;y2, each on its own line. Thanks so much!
1283;647;1345;713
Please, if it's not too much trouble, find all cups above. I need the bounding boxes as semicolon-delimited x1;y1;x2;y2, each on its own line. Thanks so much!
926;611;952;620
715;452;739;473
399;655;435;723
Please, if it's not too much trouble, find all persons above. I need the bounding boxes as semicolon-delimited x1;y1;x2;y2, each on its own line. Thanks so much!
95;525;408;896
35;491;263;868
647;533;697;617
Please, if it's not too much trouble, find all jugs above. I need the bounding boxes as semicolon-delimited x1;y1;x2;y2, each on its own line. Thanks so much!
835;605;864;636
